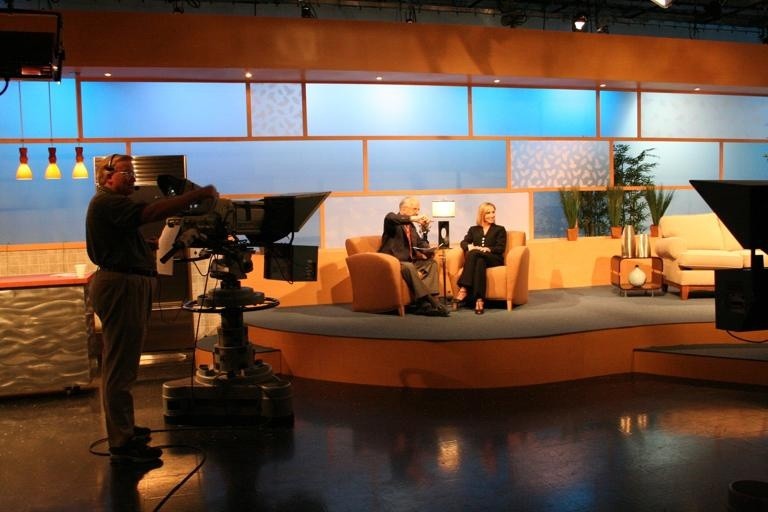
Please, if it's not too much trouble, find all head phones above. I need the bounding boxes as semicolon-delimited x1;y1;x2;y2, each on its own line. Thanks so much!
103;154;118;176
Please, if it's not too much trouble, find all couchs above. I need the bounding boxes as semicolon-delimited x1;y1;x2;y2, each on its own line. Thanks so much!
657;214;768;300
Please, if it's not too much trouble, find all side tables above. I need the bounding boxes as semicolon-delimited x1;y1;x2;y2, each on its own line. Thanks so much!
610;256;663;297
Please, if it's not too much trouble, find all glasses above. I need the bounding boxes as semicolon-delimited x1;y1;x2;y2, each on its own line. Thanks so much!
112;170;135;177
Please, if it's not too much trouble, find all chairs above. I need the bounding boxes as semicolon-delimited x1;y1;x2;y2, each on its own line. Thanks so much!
447;229;530;311
345;235;440;317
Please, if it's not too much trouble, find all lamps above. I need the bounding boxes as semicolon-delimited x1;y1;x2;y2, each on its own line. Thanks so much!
15;76;32;181
73;79;88;178
44;81;61;179
432;200;455;308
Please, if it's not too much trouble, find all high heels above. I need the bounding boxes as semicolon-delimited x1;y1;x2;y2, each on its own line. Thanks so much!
452;290;468;303
475;300;485;315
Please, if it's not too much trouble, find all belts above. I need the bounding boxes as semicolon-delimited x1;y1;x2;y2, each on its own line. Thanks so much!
96;264;159;277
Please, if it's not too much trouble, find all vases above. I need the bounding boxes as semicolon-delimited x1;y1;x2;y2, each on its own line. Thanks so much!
638;234;651;258
621;225;635;258
628;265;646;287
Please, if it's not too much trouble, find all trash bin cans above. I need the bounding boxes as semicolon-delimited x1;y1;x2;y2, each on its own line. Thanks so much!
727;479;768;512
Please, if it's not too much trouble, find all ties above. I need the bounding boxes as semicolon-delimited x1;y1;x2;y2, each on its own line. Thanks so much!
405;225;414;259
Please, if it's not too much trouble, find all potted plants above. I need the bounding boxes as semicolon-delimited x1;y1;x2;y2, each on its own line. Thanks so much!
643;181;676;237
558;184;580;241
606;182;625;239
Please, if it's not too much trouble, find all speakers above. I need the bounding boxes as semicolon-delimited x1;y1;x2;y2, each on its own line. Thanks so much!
0;29;66;82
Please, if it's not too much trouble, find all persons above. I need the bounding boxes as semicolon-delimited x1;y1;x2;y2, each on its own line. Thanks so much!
377;197;452;318
453;202;506;315
85;154;219;455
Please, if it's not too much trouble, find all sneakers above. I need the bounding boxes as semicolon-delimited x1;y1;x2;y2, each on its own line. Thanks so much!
107;444;162;462
132;426;152;436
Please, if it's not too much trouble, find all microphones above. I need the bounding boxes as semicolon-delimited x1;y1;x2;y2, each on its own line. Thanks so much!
134;185;140;191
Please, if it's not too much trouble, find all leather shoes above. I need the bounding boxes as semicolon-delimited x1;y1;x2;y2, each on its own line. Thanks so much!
436;304;449;317
414;302;432;315
